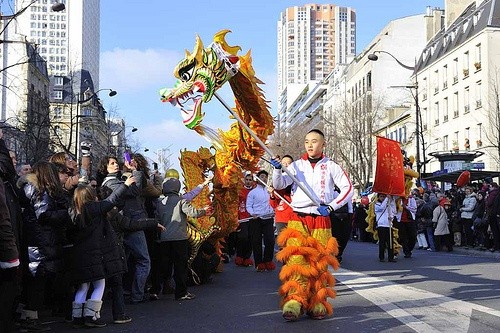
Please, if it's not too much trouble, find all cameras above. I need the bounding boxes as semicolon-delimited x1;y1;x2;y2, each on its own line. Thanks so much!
86;143;93;148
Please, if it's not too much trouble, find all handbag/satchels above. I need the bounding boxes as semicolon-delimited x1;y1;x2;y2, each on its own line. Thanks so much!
433;222;437;229
373;222;377;231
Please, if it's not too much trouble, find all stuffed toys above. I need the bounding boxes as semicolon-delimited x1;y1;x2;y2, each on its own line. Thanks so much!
365;150;420;256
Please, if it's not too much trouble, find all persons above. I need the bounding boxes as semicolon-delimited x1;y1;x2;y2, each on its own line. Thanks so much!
271;129;354;318
227;154;352;262
394;195;417;258
37;175;135;327
430;198;454;251
0;129;213;333
246;170;276;271
409;176;500;252
460;186;478;245
150;176;211;299
373;193;397;263
235;173;256;266
350;201;376;244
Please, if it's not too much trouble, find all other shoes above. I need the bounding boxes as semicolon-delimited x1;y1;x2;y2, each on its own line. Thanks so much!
64;313;72;322
21;322;51;333
418;246;428;250
404;255;410;258
380;258;385;262
264;261;275;270
307;306;325;319
282;302;303;321
14;316;44;327
234;256;243;266
256;262;265;271
489;248;495;253
388;258;397;263
52;308;66;317
243;259;253;266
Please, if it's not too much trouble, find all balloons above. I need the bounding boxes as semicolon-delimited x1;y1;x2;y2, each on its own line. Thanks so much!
457;171;470;187
361;196;369;205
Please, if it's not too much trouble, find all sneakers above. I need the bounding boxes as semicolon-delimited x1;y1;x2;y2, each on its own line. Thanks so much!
72;318;85;328
175;291;195;300
113;315;132;323
84;316;106;327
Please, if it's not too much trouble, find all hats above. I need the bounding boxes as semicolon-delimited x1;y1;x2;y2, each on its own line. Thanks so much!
431;198;438;205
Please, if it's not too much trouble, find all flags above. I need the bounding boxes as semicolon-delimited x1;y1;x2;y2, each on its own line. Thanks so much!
372;136;405;197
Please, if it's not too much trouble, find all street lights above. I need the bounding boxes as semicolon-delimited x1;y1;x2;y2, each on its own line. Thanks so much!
128;144;149;163
305;109;338;166
73;88;118;160
107;119;138;161
367;50;421;187
